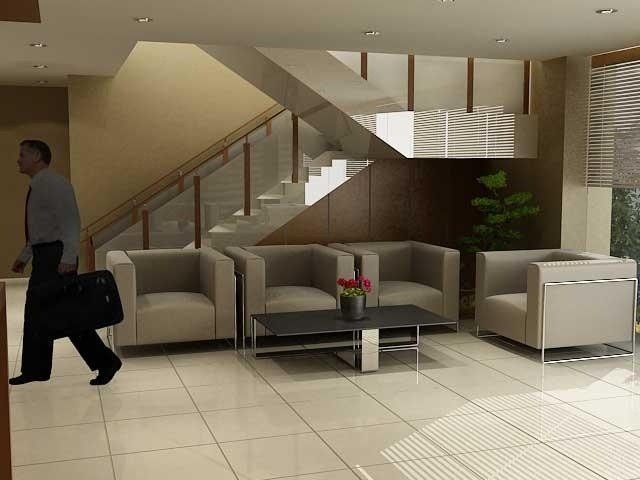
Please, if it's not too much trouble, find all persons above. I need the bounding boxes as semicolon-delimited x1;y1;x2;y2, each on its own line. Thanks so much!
7;136;123;386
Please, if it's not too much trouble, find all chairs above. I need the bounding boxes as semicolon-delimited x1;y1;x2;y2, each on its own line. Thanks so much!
224;244;355;349
327;241;460;346
474;249;638;363
106;247;237;352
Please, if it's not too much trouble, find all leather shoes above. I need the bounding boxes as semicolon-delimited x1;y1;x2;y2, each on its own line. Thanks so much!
8;370;51;386
89;354;124;385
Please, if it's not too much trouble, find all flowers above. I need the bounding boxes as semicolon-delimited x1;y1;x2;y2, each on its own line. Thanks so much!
336;276;372;296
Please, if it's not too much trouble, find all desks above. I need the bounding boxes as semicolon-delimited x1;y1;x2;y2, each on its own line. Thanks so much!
249;304;457;373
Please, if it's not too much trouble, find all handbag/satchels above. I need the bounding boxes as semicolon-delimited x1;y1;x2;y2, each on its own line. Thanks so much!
50;268;126;331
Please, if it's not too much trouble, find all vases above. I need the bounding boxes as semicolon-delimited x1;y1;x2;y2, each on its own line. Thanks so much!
341;293;365;321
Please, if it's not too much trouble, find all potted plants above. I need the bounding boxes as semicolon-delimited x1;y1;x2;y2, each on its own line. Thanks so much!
459;168;543;317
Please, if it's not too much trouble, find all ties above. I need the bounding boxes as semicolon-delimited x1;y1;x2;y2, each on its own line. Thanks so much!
24;185;33;248
